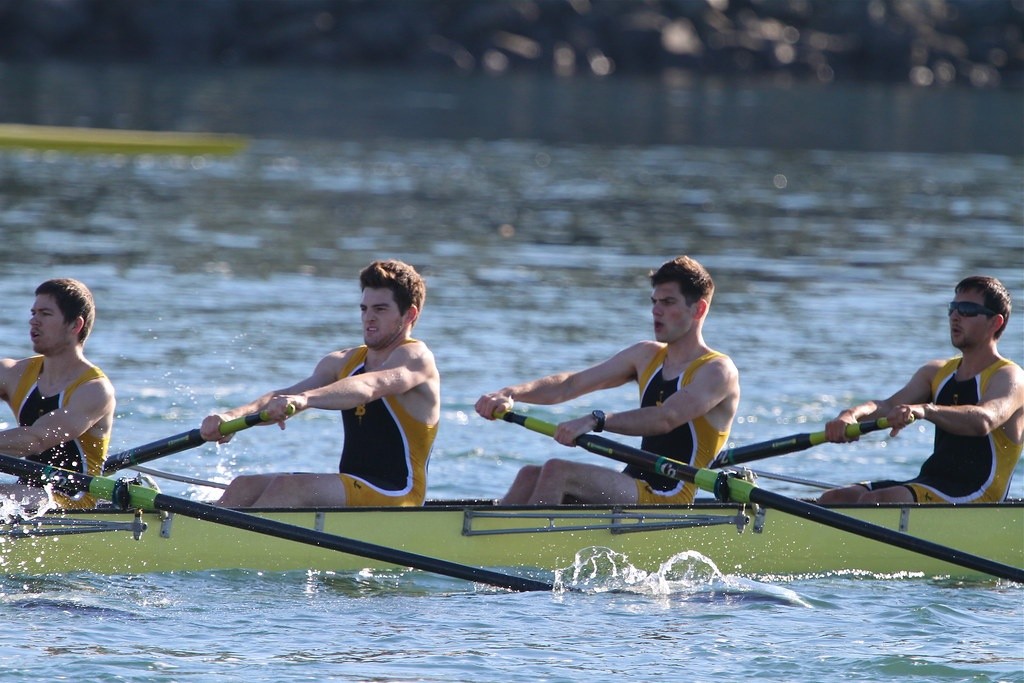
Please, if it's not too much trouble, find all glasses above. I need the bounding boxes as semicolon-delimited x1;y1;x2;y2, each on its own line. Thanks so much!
948;300;998;317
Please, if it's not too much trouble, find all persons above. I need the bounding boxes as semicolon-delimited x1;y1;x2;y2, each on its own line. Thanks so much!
200;258;441;512
474;257;741;508
0;279;117;514
743;274;1023;505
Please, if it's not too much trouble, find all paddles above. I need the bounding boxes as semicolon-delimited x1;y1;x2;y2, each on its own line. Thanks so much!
706;410;919;470
0;450;562;593
104;402;297;478
492;410;1024;588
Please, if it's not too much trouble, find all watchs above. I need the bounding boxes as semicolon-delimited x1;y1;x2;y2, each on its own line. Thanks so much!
592;411;607;433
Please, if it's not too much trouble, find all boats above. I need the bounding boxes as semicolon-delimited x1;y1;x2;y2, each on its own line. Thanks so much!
1;497;1024;597
